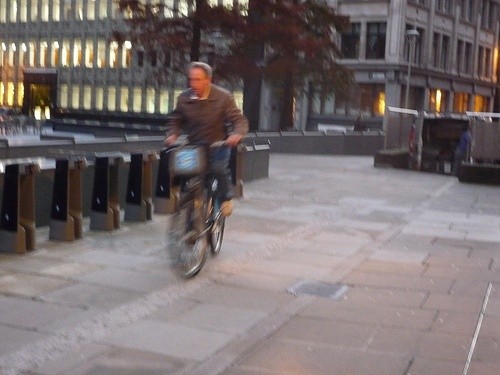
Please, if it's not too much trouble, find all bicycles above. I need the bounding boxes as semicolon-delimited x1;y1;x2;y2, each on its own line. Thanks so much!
164;136;226;279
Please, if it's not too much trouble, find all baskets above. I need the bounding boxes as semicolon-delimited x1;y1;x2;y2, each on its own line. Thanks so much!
169;146;204;175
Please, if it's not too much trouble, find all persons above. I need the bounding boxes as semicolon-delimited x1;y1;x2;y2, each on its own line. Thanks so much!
162;61;250;247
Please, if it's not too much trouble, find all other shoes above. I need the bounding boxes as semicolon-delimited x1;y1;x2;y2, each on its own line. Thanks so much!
222;201;232;216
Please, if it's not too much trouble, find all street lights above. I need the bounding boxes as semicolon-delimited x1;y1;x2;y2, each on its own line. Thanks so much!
404;30;421;108
